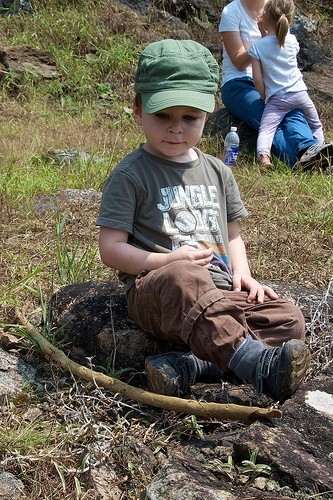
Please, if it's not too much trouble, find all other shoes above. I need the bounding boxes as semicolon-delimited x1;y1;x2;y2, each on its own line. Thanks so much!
257;157;272;175
299;142;332;172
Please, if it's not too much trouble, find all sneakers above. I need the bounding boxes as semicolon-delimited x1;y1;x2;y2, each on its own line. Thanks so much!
142;351;199;397
253;338;311;407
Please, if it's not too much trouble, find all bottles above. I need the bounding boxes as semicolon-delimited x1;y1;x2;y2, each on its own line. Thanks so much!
222;127;240;164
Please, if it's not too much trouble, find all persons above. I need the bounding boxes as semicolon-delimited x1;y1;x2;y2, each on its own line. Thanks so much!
219;0;333;173
95;38;311;405
248;0;325;167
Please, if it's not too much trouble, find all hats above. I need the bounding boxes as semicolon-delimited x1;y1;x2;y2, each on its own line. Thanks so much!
133;39;219;115
